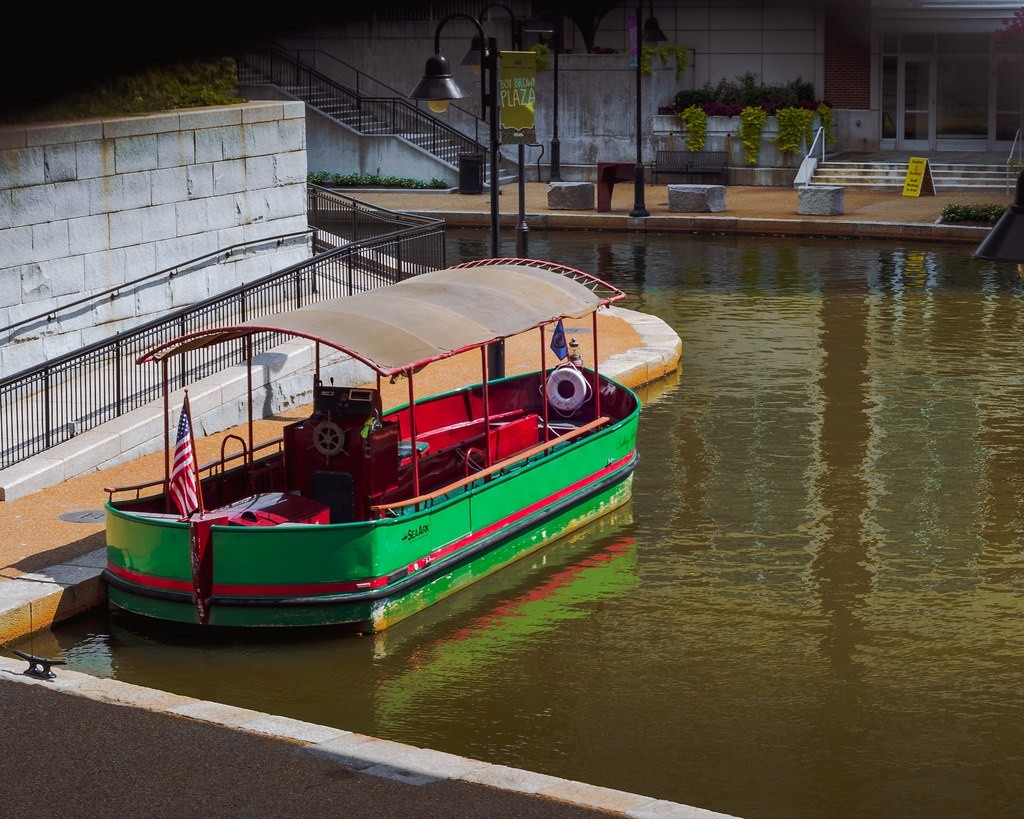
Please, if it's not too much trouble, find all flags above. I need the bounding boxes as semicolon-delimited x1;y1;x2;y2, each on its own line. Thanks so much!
549;318;570;362
169;389;206;521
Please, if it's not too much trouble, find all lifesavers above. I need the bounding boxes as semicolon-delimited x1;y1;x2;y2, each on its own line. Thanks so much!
543;368;588;412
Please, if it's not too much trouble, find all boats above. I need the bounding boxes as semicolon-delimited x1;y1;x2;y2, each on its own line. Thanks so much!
100;256;645;636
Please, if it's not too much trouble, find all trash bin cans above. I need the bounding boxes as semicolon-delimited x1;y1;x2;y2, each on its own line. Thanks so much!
457;152;483;192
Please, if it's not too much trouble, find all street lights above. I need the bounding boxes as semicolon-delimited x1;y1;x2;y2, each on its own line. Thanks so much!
626;0;671;217
405;11;500;258
521;0;563;185
457;3;534;260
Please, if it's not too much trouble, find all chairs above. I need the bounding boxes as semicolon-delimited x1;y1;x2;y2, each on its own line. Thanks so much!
388;413;430;476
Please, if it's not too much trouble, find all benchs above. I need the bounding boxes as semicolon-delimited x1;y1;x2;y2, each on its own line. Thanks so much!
465;415;539;477
650;150;729;187
